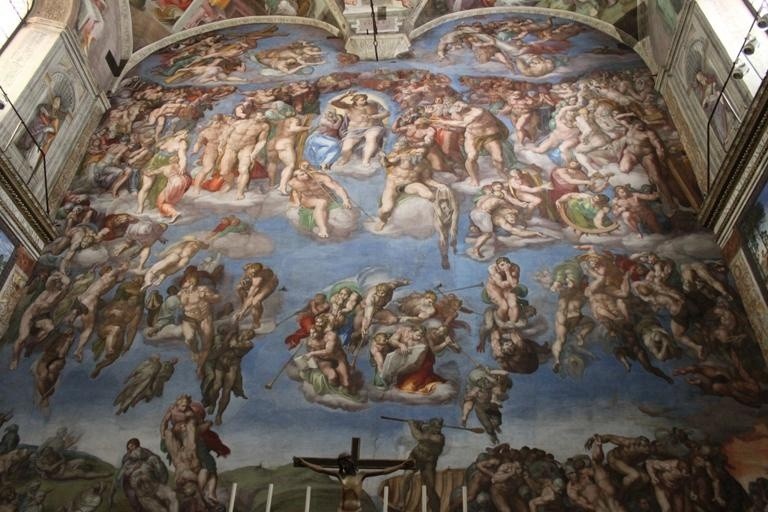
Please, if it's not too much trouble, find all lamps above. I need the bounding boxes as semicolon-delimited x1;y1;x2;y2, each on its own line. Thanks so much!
728;2;768;83
